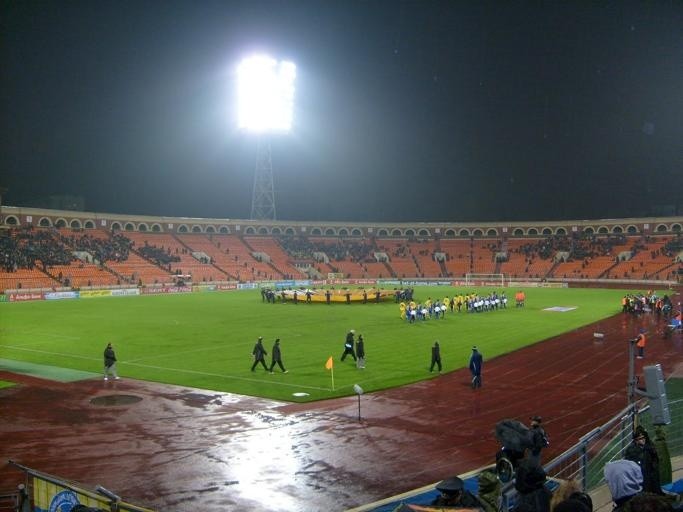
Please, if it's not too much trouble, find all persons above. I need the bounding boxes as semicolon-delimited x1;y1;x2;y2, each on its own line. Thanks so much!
356;334;366;369
635;327;646;359
341;329;356;363
429;415;683;512
250;337;270;372
430;341;443;374
469;346;483;388
268;338;288;374
103;343;120;381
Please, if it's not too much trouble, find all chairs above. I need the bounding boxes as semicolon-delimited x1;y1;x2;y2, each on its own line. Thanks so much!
333;233;682;280
1;225;332;291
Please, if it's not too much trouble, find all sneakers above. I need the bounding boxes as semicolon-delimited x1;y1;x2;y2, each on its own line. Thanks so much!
268;370;275;375
103;377;108;381
281;370;289;374
112;376;120;380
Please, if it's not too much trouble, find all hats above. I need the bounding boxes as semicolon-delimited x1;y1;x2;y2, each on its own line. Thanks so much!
435;475;464;500
